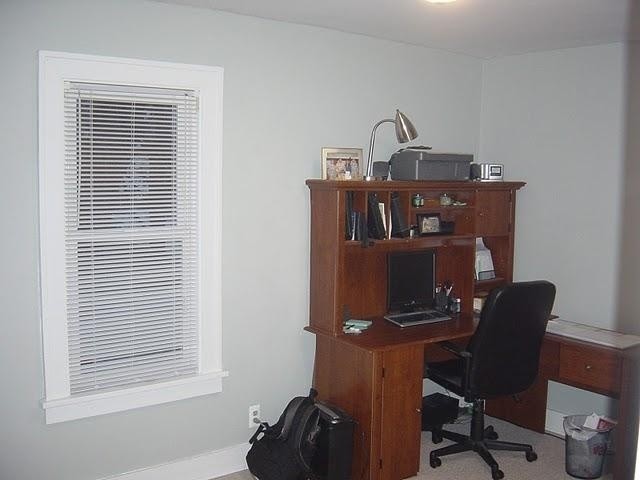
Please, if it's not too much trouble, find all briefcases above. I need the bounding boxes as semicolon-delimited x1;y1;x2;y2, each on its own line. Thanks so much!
313;400;355;480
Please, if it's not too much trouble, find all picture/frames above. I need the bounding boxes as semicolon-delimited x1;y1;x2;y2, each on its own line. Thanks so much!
321;147;364;179
418;213;442;236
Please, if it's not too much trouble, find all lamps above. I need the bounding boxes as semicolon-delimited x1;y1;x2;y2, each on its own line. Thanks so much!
364;109;418;179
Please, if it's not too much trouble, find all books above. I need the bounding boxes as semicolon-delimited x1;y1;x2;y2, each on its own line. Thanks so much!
345;319;372;327
343;190;407;241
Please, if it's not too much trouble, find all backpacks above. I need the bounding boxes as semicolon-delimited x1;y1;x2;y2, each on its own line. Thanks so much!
246;388;322;480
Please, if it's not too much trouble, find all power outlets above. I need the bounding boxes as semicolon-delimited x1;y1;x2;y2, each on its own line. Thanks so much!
249;405;260;430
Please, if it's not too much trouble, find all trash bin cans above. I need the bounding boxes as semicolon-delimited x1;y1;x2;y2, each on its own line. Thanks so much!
563;414;618;479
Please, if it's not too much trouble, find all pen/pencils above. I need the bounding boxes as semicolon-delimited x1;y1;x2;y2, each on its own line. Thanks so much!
436;280;455;297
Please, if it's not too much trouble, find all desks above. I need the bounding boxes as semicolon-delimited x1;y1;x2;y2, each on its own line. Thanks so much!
304;180;640;480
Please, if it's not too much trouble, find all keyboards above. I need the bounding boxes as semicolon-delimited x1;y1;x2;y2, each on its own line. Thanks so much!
384;309;452;328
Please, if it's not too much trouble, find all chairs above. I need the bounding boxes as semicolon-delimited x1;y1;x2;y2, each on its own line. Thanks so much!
424;281;556;480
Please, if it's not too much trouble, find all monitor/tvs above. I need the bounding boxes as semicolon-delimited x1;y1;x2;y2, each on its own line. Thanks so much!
387;250;436;313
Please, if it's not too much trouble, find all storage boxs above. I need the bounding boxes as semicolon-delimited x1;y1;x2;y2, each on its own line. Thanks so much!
391;153;473;181
